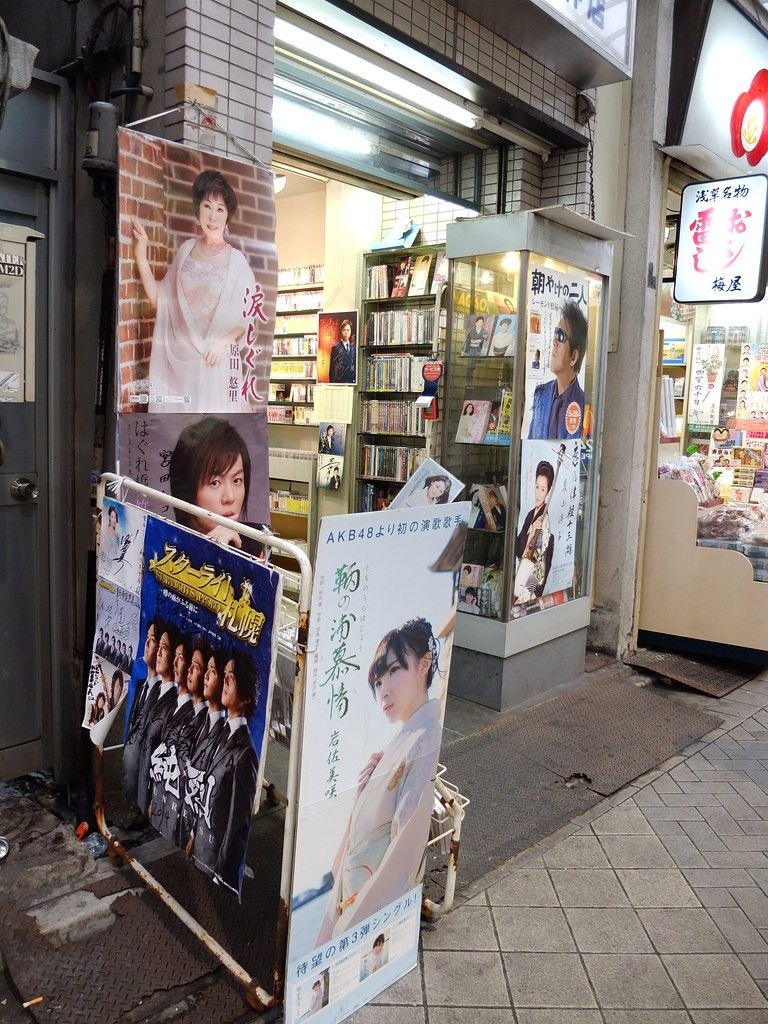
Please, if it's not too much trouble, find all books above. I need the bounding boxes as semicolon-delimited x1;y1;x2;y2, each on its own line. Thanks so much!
266;264;322;517
457;562;503;619
465;482;507;532
454;384;512;447
355;251;451;514
461;314;518;357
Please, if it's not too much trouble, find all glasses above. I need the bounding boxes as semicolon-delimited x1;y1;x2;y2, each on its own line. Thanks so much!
554;327;573;344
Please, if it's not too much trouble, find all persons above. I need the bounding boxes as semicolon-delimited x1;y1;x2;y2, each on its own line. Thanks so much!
492;318;514;355
486;403;502;434
411;255;429;291
313;312;356;491
396;256;410;275
392;475;451;510
169;417;267;560
462;316;489;357
484;574;495;589
457;586;480;615
434;253;449;282
83;505;447;1018
511;297;768;626
133;169;257;413
488;490;507;531
461;565;472;585
468;489;480;506
460;403;478;437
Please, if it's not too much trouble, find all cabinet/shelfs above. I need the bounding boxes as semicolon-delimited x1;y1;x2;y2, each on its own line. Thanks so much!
348;244;454;515
266;285;319;576
446;214;613;658
